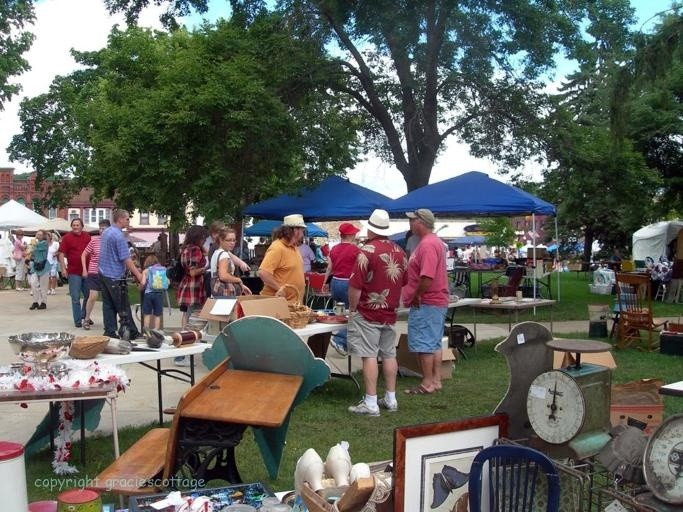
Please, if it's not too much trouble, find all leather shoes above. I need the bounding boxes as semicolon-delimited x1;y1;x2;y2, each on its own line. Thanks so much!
38;303;46;309
75;321;82;328
29;302;38;310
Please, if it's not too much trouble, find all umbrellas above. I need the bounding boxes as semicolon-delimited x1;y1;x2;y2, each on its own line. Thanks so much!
445;234;500;264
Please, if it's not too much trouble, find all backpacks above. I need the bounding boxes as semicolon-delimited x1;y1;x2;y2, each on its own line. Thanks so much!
146;263;169;291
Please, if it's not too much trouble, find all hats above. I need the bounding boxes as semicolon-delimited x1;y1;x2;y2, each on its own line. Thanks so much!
280;214;308;229
360;208;395;236
404;207;434;229
338;222;360;235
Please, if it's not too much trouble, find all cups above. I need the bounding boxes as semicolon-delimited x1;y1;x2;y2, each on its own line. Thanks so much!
333;302;346;317
515;290;523;301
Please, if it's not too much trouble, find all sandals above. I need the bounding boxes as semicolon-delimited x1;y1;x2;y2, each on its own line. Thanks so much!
88;318;94;325
404;384;434;395
82;320;90;329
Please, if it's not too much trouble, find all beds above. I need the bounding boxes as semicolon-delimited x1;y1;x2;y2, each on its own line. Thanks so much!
11;334;213;468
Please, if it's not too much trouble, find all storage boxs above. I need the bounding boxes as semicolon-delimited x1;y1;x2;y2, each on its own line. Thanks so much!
196;294;292;335
609;377;664;436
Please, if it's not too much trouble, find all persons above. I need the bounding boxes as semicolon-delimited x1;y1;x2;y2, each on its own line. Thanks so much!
345;207;410;417
607;249;622;272
400;207;449;395
446;244;517;266
178;214;364;356
404;235;420;262
8;208;170;342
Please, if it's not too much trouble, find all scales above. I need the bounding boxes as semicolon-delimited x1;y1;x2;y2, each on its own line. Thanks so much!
527;339;612;462
637;378;683;512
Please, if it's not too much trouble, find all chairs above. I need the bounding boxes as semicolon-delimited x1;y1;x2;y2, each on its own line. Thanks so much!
446;265;473;324
481;264;526;314
304;271;332;312
469;444;561;512
613;269;669;353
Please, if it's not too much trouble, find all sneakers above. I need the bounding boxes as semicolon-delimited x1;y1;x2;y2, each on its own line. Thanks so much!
378;398;398;412
16;287;24;290
348;404;381;418
30;289;33;296
47;287;56;296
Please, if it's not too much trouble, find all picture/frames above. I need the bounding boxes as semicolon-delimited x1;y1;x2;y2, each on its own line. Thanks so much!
393;413;510;512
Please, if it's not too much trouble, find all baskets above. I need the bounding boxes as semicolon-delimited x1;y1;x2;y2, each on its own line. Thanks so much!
588;282;614;296
528;247;547;260
588;303;610;324
668;314;683;333
272;284;313;328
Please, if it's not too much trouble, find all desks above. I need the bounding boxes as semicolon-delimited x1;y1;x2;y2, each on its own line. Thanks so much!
659;380;683;397
378;297;482;376
198;319;361;396
0;372;128;509
521;272;554;308
470;295;557;354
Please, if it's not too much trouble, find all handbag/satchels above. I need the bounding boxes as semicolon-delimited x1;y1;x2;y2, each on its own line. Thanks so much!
32;259;47;272
203;268;211;298
212;251;243;298
166;250;184;283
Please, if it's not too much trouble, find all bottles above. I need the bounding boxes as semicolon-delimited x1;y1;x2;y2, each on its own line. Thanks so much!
0;440;103;512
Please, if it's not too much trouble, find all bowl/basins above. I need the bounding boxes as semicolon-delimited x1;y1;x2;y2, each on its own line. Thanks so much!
7;332;74;361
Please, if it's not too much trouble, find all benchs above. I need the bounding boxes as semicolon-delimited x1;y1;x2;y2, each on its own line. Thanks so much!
85;356;304;512
